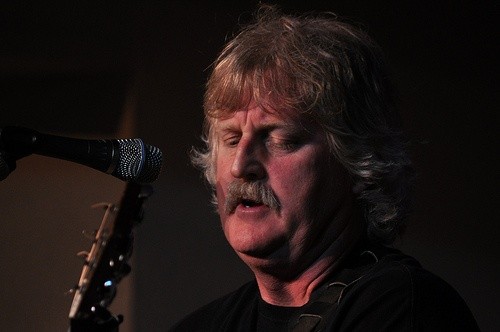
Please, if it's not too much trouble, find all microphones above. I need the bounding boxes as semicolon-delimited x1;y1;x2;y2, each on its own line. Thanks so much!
0;125;164;185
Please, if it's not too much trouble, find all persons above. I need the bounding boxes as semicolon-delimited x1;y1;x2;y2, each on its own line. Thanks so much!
161;8;499;330
